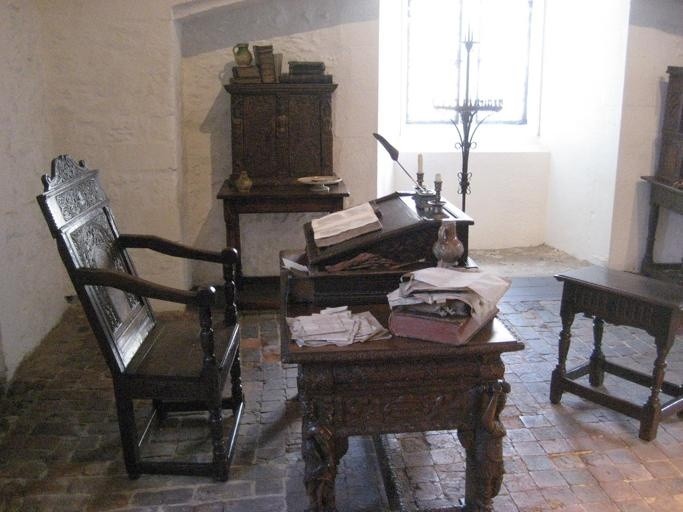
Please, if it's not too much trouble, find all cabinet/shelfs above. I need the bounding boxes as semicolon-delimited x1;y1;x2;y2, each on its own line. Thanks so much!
223;84;337;186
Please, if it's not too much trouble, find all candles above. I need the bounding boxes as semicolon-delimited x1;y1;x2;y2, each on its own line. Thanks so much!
416;153;424;173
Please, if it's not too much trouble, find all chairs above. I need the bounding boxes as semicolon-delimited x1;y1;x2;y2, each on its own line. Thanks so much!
37;152;247;485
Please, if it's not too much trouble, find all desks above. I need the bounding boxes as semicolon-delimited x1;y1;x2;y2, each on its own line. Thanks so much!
277;296;523;512
277;246;483;309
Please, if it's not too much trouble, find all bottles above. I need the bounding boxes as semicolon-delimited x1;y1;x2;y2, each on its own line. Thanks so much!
234;171;252;191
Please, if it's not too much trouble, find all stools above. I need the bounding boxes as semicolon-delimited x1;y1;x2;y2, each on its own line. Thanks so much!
549;267;681;443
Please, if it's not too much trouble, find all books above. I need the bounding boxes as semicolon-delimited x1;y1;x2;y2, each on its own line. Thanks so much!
311;202;382;249
287;267;513;348
